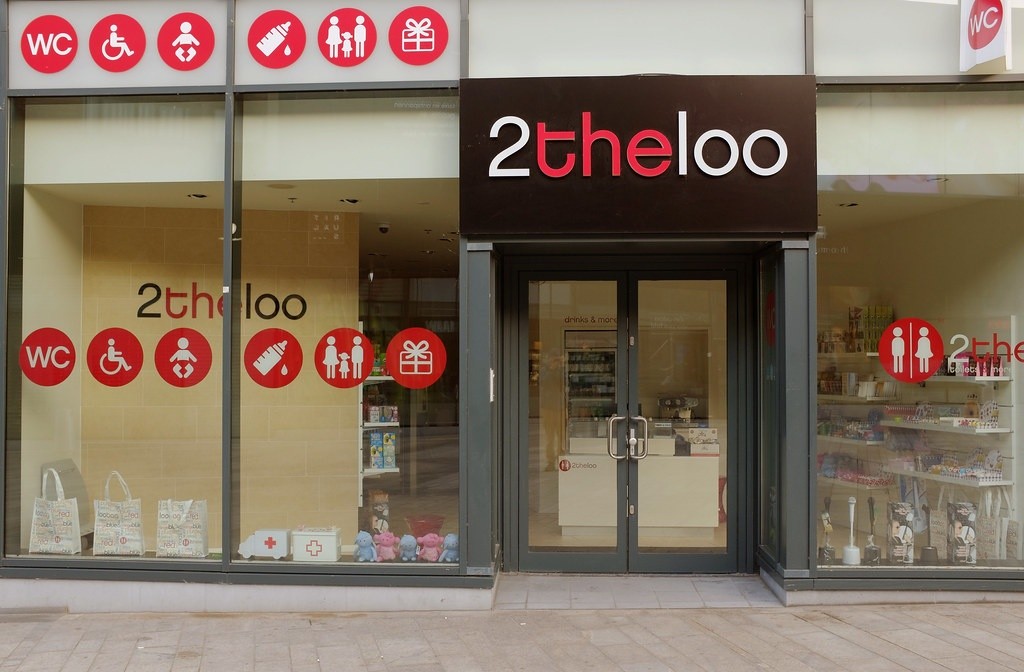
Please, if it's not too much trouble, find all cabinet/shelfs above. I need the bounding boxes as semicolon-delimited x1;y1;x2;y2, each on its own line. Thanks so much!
814;314;1017;560
565;348;618;418
358;321;399;507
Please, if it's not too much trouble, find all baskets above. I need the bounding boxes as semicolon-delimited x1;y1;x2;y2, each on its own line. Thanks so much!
405;515;445;538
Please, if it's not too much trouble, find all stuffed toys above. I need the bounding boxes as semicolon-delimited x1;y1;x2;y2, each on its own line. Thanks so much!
353;531;460;565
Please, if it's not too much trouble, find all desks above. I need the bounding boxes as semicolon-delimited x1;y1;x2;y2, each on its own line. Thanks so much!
558;437;719;528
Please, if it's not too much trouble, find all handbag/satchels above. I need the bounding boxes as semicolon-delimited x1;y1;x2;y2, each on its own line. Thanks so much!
93;471;144;555
28;468;81;555
155;499;208;557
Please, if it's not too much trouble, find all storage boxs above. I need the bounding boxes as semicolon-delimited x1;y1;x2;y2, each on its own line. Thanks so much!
292;525;342;561
255;529;291;557
